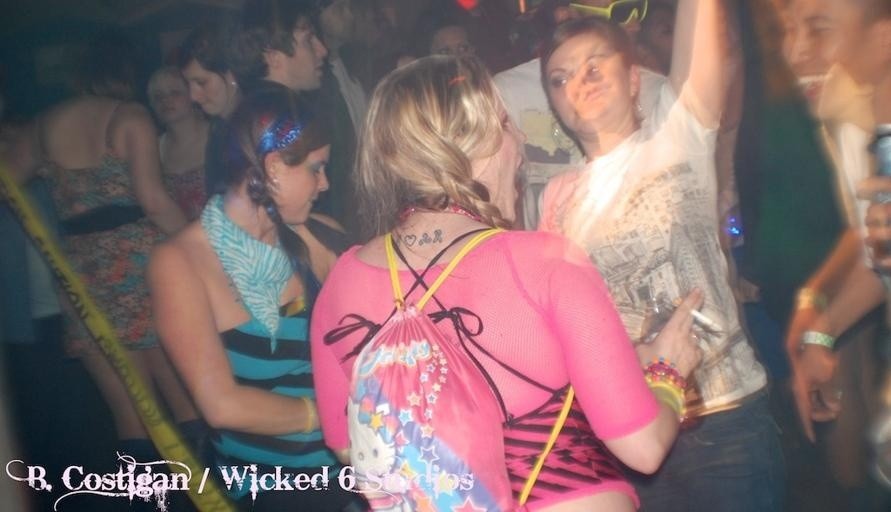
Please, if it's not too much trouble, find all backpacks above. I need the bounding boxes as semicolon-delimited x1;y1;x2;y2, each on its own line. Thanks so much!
343;226;586;512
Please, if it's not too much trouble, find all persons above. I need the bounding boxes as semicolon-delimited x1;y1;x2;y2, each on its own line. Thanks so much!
0;1;891;511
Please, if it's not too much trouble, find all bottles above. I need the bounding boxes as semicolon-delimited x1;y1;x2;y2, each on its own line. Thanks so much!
644;296;703;433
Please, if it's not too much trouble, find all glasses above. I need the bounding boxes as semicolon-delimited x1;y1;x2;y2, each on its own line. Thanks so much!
570;0;649;29
